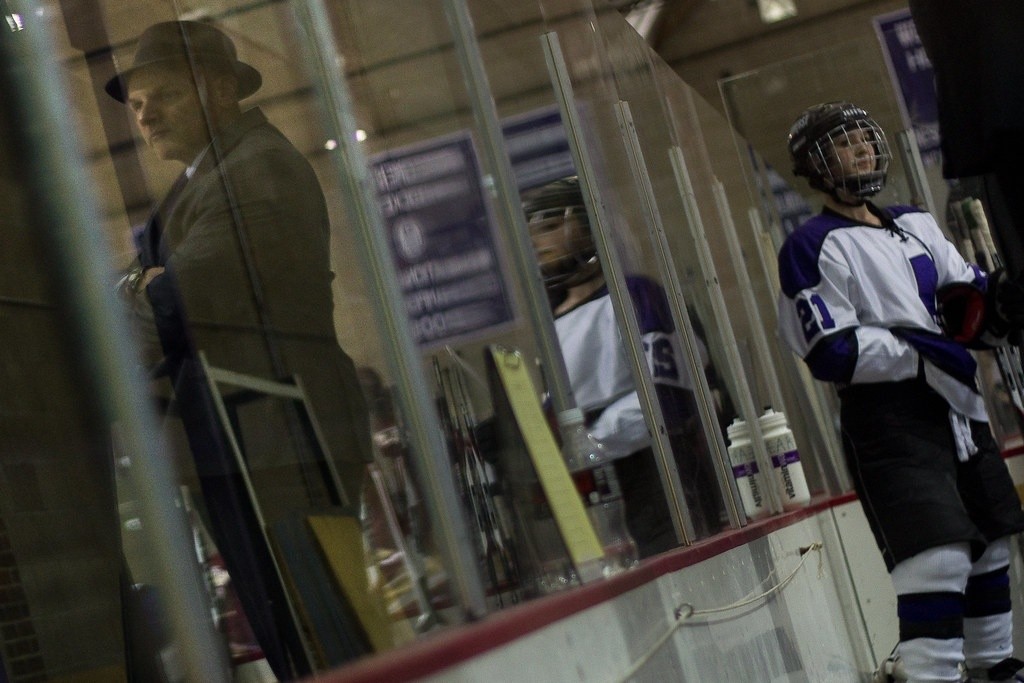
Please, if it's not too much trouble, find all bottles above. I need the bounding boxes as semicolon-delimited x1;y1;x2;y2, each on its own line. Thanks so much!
757;406;810;510
727;417;770;518
530;472;574;592
555;409;637;576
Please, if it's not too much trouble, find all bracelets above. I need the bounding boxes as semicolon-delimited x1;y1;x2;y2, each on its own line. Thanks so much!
103;19;377;683
126;267;145;298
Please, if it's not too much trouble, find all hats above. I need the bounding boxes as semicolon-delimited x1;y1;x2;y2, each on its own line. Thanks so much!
105;20;263;103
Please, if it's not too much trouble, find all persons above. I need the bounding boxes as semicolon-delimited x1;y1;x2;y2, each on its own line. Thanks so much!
773;91;1022;683
520;172;730;566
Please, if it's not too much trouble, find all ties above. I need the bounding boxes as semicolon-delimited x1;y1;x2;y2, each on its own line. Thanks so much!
169;167;190;203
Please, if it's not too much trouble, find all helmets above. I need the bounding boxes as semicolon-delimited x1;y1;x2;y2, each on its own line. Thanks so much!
785;100;894;198
520;175;606;289
